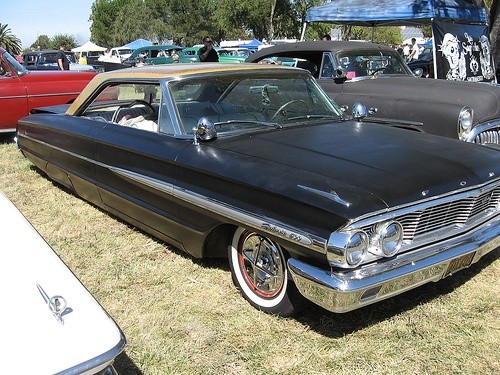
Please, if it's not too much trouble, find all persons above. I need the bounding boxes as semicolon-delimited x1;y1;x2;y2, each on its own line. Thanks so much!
70;52;89;64
388;43;425;61
57;42;69;70
407;38;419;61
199;36;219;62
323;35;331;41
172;54;182;65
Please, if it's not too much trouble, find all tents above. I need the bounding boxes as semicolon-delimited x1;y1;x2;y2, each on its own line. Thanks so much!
301;0;498;84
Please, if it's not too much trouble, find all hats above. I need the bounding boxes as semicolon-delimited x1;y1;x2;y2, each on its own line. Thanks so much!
59;41;67;46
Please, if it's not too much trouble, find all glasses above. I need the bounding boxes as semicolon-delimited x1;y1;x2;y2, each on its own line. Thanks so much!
205;40;211;43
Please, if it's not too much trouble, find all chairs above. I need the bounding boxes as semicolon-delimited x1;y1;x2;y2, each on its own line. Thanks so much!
99;102;267;134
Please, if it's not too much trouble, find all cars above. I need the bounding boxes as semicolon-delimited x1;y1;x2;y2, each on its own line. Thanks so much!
0;47;120;134
21;45;297;86
14;59;500;322
217;39;500;146
